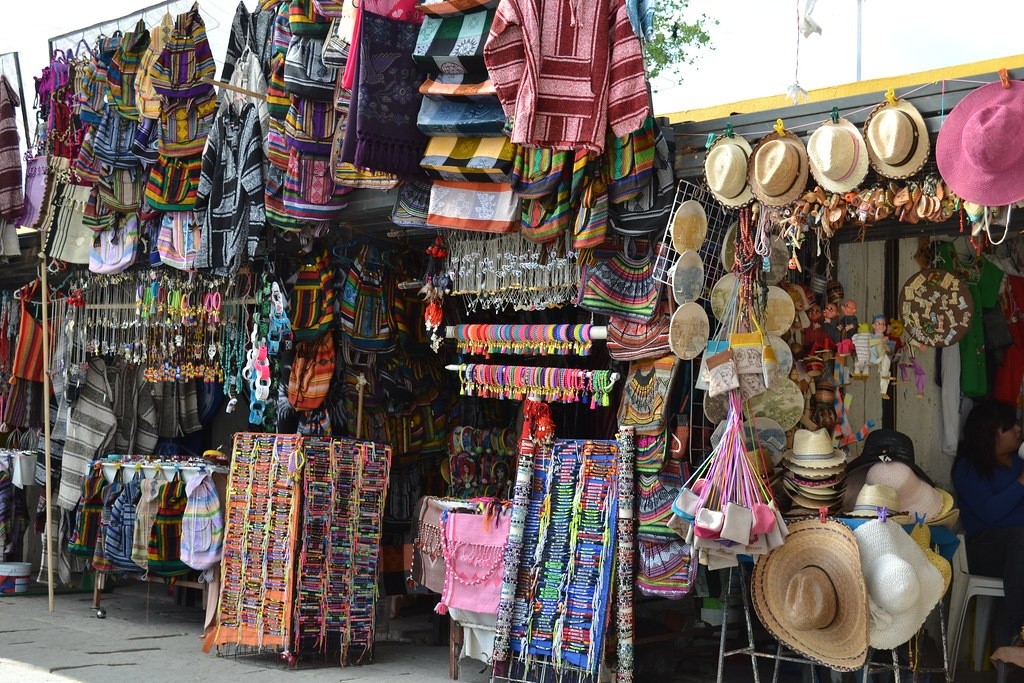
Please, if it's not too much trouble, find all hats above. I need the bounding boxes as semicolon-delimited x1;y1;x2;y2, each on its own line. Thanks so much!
750;517;869;673
702;132;755;209
807;118;868;194
853;519;944;650
742;427;959;530
909;523;952;598
936;80;1024;206
746;129;809;209
863;98;930;179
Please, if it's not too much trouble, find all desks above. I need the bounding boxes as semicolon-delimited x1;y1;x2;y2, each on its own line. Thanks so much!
423;497;513;681
90;464;227;616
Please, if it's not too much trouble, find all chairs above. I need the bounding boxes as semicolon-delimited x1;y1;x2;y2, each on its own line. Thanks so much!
946;527;1006;683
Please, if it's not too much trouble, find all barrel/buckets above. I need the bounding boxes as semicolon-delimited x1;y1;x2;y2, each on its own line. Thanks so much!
0;562;33;594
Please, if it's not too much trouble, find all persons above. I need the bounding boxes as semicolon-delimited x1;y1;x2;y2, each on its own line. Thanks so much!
852;322;874;380
887;317;927;399
441;424;519;483
819;303;842;359
796;303;824;363
951;393;1024;646
871;315;897;399
837;299;859;357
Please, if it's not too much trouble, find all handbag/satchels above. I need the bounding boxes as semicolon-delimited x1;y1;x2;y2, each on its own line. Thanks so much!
65;466;224;587
634;539;698;601
13;2;673;438
636;459;692;544
1;429;42;553
667;267;788;570
633;436;667;473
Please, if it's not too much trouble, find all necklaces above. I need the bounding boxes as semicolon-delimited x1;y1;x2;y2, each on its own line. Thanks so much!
726;201;770;276
418;236;579;314
48;269;245;415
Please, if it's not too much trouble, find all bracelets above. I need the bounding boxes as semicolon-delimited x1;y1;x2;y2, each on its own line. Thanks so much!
451;322;615;411
244;285;293;426
492;429;636;682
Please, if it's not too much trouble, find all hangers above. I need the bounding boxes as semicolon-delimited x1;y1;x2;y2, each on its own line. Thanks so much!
14;256;79;304
52;0;220;62
954;234;971;257
0;54;4;74
332;232;400;269
24;110;47;161
